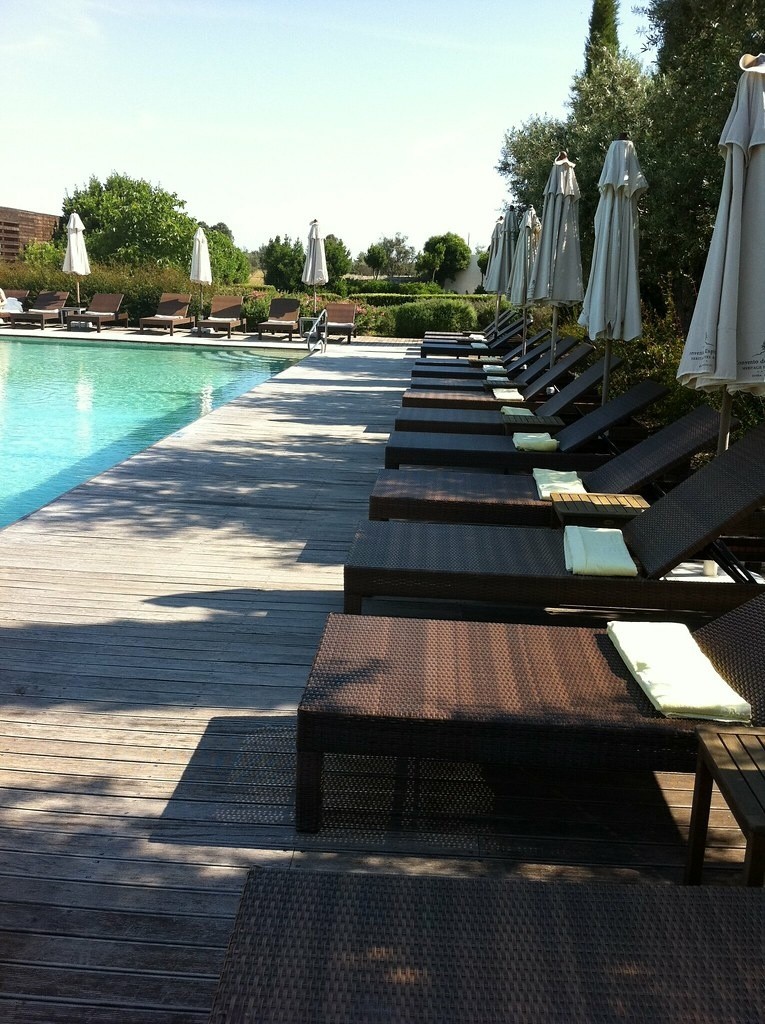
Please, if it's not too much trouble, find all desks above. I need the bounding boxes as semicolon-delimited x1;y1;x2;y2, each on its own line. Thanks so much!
501;415;566;474
550;492;651;527
462;330;485;336
468;359;504;367
456;337;483;359
482;380;527;392
60;307;86;329
298;316;319;339
683;724;765;887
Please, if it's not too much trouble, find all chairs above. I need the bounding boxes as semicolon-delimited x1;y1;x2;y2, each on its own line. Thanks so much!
298;592;765;837
206;868;765;1024
317;303;357;344
139;293;195;336
342;418;765;619
197;295;246;338
67;294;128;333
9;291;70;330
258;298;300;341
0;289;30;317
369;307;742;526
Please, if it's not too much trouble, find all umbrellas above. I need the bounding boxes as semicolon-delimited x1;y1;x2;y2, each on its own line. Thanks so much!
577;131;649;439
301;221;330;331
484;152;584;371
677;53;765;458
188;229;218;325
62;210;90;328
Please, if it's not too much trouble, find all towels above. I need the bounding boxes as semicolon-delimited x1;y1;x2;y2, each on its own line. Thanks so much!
154;315;183;319
469;334;487;341
512;433;560;451
471;343;488;348
492;388;524;401
268;320;295;324
607;619;752;724
326;322;355;327
487;376;509;381
501;406;534;416
208;316;239;321
86;311;115;316
483;365;507;371
29;309;58;314
533;468;587;501
563;526;638;576
